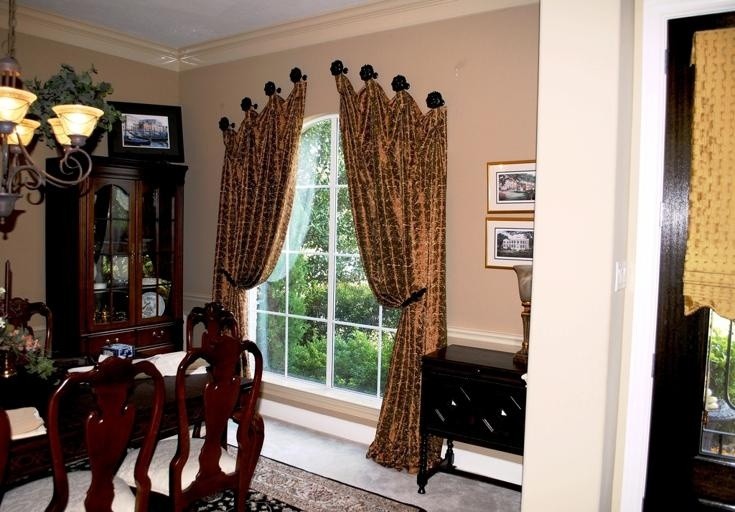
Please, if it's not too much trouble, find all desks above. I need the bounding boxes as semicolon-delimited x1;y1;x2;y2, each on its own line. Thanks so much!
0;350;259;463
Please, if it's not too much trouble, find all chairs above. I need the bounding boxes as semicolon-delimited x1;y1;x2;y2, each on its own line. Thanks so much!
117;332;264;512
186;302;243;440
0;297;55;483
0;357;170;511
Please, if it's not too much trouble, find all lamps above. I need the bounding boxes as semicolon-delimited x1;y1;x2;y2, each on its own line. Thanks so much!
0;0;104;226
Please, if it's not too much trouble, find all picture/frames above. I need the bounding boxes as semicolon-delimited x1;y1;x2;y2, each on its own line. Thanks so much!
107;101;184;162
485;158;536;270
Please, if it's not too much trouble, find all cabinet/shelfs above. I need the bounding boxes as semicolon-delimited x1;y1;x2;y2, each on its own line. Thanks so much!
417;345;526;494
45;155;190;359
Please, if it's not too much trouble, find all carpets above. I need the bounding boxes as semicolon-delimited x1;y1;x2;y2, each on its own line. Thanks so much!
0;436;427;512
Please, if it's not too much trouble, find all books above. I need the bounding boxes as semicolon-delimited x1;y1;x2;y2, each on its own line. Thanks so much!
5;405;47;440
